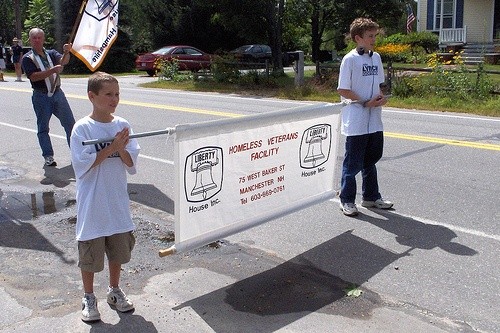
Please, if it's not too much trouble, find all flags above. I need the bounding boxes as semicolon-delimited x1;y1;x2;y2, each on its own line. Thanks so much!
407;7;416;33
70;0;118;72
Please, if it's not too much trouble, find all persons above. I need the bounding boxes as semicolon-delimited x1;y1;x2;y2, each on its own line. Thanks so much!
21;28;75;166
0;39;7;82
338;18;394;216
9;37;23;82
70;70;141;322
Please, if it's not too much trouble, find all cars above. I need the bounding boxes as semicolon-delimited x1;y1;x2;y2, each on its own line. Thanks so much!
135;44;215;78
228;44;294;66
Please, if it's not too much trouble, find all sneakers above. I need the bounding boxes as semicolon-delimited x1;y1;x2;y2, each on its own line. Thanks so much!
106;285;135;310
81;292;101;321
361;197;394;208
340;202;359;215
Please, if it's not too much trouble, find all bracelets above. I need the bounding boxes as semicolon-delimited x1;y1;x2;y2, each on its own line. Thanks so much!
363;100;369;108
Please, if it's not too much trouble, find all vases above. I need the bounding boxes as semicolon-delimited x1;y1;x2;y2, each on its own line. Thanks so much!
440;53;454;64
483;53;500;65
446;44;462;54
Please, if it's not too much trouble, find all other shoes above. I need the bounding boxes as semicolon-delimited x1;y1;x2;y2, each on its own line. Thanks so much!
45;156;57;165
